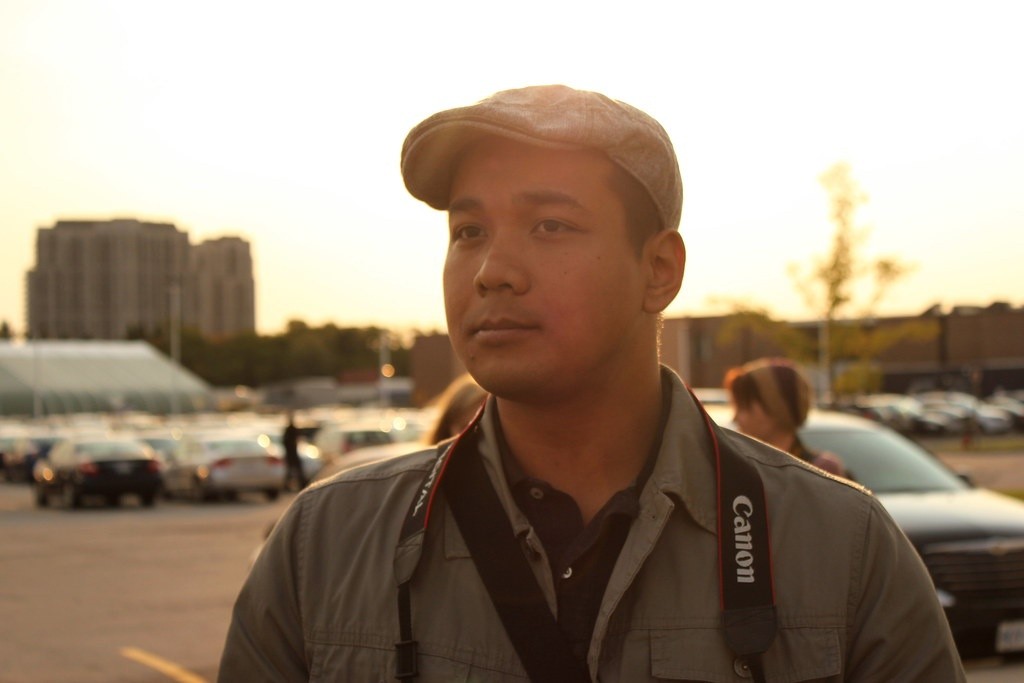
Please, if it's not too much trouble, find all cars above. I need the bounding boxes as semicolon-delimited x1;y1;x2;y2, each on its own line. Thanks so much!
701;402;1024;664
0;391;1023;510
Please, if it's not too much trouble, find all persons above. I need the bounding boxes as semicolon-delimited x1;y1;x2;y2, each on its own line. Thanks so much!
281;413;324;490
212;82;969;683
723;361;859;485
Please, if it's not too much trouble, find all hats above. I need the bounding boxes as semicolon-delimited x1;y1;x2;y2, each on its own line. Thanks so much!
400;85;682;230
